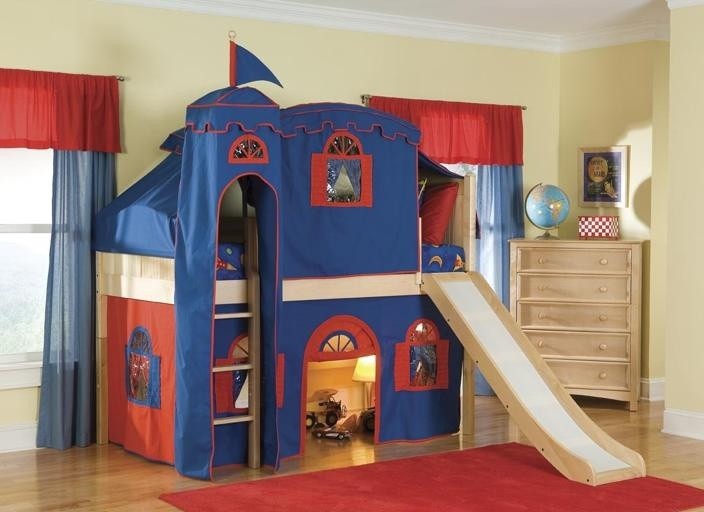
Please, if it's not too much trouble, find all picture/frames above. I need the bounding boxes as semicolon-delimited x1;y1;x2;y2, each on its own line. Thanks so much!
576;144;631;207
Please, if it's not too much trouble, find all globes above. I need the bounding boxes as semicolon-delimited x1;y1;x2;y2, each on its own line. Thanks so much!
524;183;570;240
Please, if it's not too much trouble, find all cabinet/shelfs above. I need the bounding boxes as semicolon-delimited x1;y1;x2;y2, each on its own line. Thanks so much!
506;236;642;412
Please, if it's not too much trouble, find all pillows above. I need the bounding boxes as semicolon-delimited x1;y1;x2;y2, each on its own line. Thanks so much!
416;181;461;247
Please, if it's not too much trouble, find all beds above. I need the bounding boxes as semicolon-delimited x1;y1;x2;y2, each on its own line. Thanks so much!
92;217;471;284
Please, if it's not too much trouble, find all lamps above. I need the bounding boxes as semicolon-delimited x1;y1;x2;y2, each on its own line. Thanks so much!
351;354;376;412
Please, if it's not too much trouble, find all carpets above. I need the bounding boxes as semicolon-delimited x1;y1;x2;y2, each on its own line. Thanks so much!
158;442;703;512
